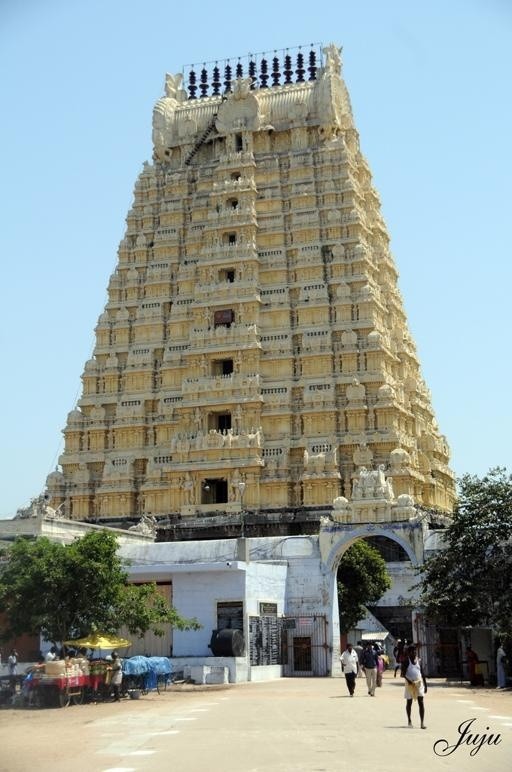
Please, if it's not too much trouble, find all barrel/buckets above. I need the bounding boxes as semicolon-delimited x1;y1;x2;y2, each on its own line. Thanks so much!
208;628;245;656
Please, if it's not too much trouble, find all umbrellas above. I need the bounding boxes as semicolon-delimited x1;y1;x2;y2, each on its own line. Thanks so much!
64;630;133;658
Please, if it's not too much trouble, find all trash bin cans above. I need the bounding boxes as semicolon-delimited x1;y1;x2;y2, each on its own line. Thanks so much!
130;689;140;699
184;666;190;679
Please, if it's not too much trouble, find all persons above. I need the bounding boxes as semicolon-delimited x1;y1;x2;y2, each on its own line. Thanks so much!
38;643;89;666
339;636;427;730
465;646;484;687
8;648;20;675
495;641;509;689
107;651;123;702
